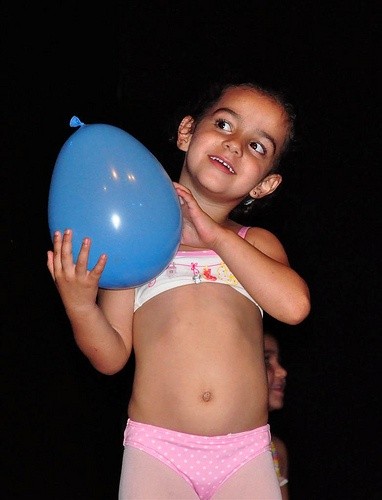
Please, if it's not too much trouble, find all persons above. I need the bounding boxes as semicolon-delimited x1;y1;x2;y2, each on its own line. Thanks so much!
259;328;291;499
46;80;311;499
